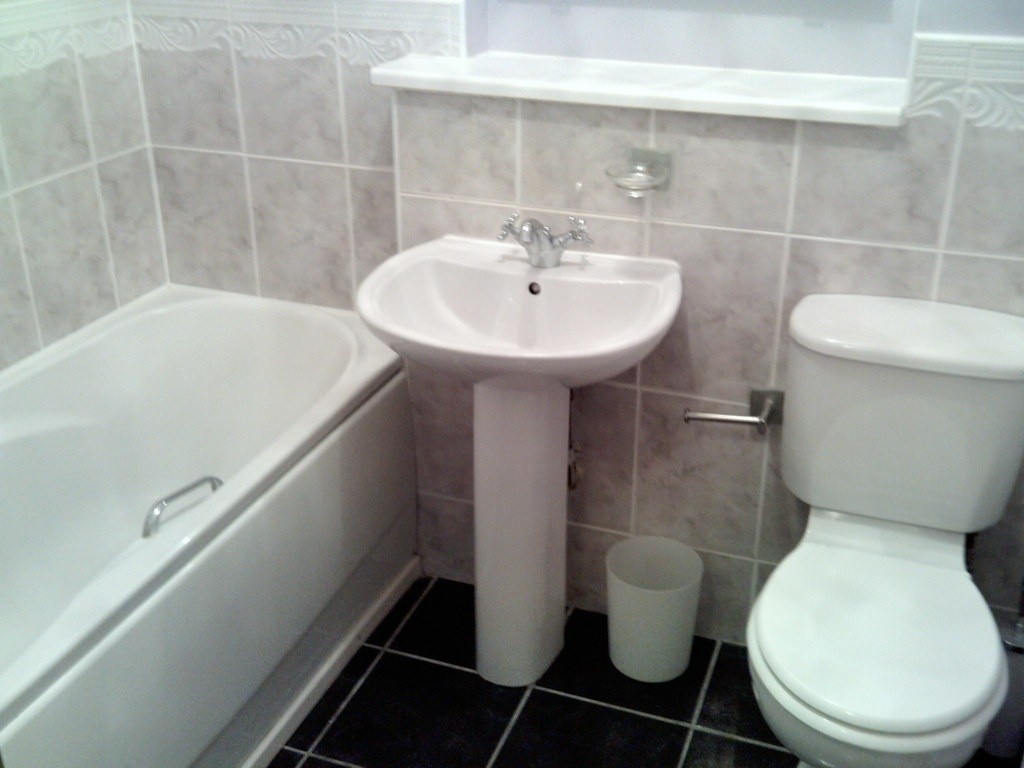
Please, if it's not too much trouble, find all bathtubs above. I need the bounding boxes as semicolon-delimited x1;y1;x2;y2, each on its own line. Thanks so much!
2;281;415;767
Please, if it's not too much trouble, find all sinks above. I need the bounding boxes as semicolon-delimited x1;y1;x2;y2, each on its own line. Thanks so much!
355;236;682;380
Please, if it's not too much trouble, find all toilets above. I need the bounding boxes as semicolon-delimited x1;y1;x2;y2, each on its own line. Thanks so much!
743;295;1024;768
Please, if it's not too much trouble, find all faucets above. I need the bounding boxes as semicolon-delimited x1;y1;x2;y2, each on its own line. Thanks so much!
501;208;591;268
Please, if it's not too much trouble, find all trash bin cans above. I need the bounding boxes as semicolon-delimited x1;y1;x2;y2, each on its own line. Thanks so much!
606;537;704;683
